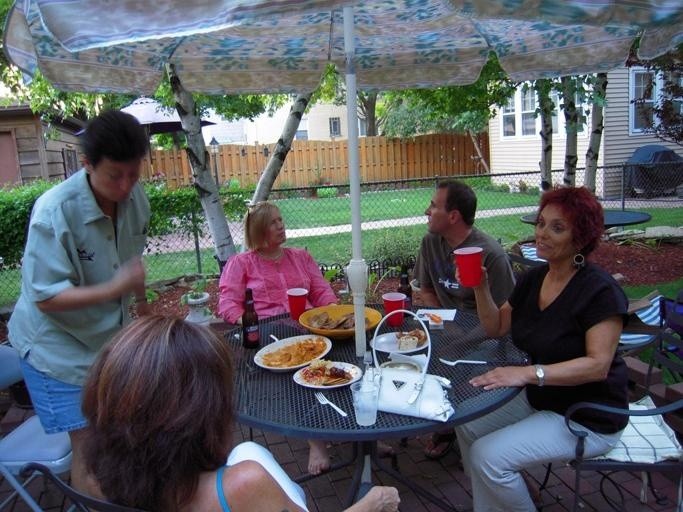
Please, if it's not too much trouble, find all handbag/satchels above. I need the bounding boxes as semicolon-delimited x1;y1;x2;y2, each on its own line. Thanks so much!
362;308;455;422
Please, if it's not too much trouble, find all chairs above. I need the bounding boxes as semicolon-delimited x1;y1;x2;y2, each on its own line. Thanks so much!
214;246;308;442
515;243;547;272
0;344;93;512
399;237;546;448
538;295;683;512
19;462;372;512
618;289;670;359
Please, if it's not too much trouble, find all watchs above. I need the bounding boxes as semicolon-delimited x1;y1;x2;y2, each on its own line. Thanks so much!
534;364;545;387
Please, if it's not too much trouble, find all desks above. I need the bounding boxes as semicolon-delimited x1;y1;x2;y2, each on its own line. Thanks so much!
520;209;652;230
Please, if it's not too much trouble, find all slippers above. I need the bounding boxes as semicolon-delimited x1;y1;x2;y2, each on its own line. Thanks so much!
426;432;457;461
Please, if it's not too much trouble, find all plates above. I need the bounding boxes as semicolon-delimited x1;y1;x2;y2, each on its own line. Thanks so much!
293;361;362;388
369;330;431;353
300;305;383;340
252;334;332;372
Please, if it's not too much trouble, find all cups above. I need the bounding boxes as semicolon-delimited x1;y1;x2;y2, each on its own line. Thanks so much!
350;381;381;427
382;292;407;327
286;287;308;322
454;247;484;289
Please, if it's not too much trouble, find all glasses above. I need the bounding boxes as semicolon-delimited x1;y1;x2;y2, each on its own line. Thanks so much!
245;201;264;220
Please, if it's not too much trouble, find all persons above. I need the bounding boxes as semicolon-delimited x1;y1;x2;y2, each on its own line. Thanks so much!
9;107;152;501
454;185;630;512
84;311;401;512
410;179;517;460
215;202;397;474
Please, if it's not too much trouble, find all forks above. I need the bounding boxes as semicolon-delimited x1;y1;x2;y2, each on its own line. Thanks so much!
313;392;348;417
439;357;488;366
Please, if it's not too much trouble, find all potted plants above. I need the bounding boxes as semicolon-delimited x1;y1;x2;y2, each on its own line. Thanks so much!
181;275;216;326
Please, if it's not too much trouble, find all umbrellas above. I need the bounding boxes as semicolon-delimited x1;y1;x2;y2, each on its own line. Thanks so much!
0;2;683;359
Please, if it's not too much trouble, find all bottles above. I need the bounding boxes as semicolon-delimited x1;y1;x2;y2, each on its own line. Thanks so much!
243;287;261;349
